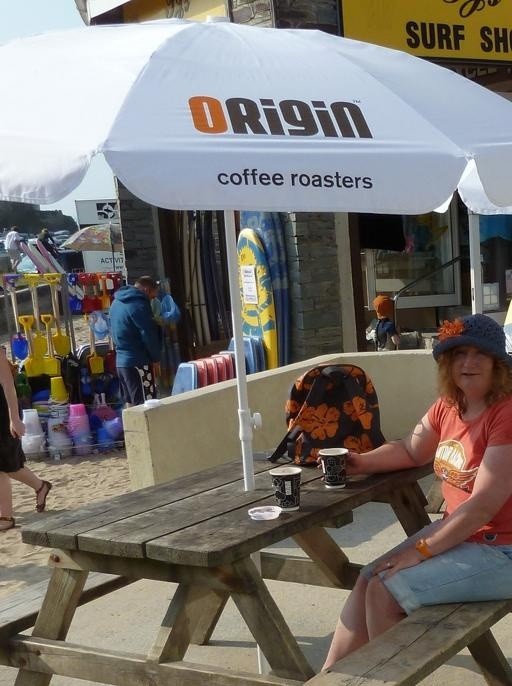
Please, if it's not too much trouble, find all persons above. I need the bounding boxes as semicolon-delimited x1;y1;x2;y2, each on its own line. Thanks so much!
5;225;21;273
317;313;512;671
365;294;401;351
36;228;56;260
0;347;52;533
109;275;162;405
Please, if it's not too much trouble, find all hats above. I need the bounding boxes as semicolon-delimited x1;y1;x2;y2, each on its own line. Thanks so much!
373;295;393;319
433;314;512;367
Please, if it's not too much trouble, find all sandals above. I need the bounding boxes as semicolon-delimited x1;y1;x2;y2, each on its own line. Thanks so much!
36;480;51;512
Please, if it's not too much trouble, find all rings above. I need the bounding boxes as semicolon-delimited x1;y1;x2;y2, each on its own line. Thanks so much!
386;562;391;567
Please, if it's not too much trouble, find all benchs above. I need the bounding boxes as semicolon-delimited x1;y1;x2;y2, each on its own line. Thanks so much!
1;565;138;686
282;585;511;686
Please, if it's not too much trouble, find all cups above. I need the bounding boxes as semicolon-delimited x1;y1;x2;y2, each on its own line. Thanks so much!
319;447;350;490
268;465;302;513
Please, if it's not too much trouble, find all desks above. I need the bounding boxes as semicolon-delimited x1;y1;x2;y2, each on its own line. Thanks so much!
17;447;512;686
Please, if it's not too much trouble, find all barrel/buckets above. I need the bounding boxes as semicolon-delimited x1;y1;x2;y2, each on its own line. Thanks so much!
97;427;117;453
47;376;69;406
67;403;94;456
20;408;48;461
47;406;74;459
102;417;124;439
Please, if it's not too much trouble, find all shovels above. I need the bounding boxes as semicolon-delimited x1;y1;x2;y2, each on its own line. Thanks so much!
4;272;122;378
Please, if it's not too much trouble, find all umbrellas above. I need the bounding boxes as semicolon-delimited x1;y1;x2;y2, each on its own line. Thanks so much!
61;221;123;272
0;14;512;670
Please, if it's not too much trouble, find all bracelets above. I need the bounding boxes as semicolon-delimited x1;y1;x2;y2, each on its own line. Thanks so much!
415;538;433;559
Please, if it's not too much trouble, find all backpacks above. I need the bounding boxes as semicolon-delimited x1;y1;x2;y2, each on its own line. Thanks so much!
268;364;386;465
